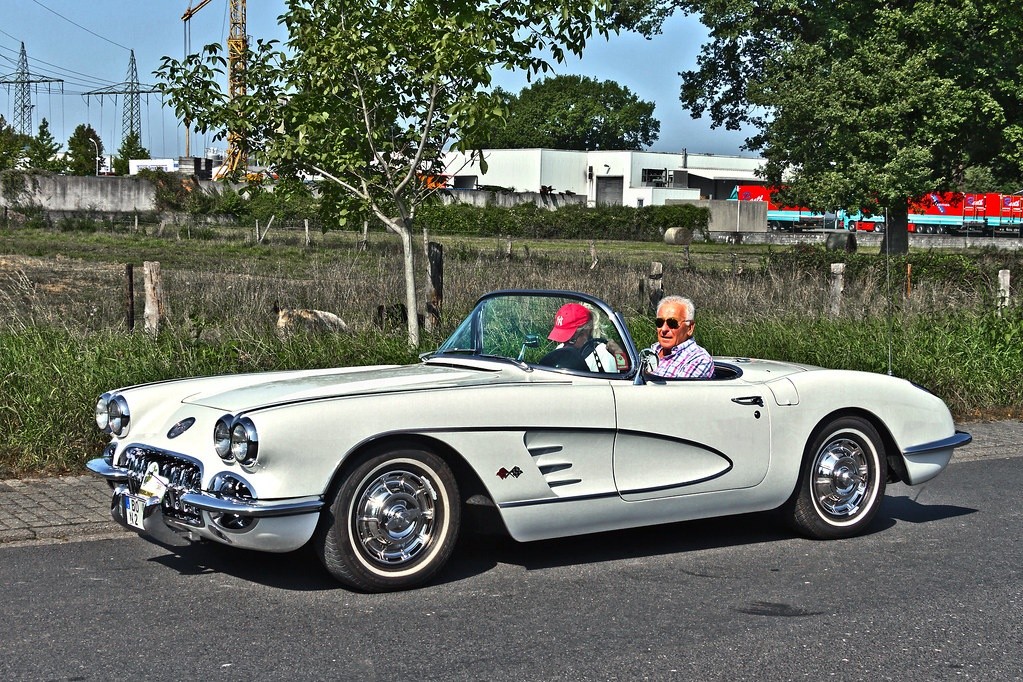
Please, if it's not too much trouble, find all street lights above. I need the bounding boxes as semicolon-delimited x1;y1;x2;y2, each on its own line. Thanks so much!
89;138;98;178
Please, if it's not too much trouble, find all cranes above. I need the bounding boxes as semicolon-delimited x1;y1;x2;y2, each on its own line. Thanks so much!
181;0;272;183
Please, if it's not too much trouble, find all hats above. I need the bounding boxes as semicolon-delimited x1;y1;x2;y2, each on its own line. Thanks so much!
548;303;591;342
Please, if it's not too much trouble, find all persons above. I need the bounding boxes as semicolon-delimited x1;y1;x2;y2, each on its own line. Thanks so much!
605;293;716;379
547;302;620;379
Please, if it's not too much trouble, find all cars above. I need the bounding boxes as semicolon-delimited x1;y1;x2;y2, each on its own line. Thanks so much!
86;291;974;597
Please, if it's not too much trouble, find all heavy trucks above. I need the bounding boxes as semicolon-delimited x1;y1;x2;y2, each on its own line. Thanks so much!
726;185;1023;237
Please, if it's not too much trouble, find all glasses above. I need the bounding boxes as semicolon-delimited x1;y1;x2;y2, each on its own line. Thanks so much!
563;324;591;344
655;317;694;329
